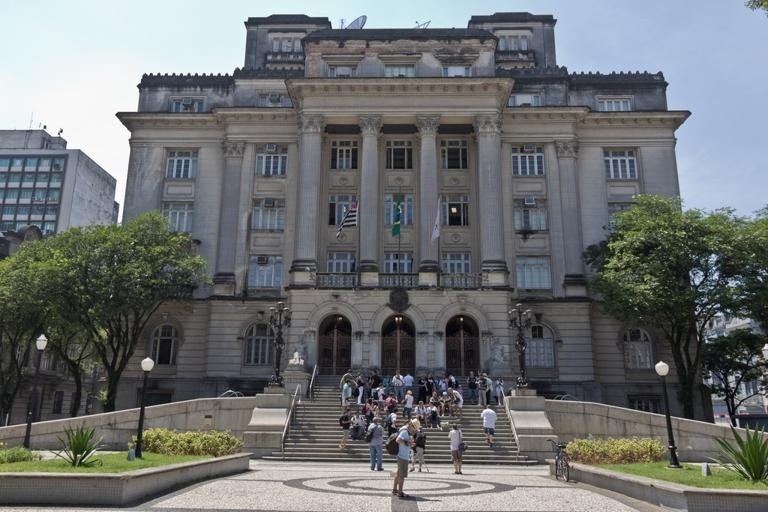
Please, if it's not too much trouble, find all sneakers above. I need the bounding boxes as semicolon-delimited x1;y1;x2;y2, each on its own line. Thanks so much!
371;467;384;470
397;492;410;498
409;468;421;472
392;490;399;495
455;471;463;474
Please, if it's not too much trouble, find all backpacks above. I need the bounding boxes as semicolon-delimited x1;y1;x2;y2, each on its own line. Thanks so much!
477;378;488;391
414;430;426;447
339;374;407;440
385;428;410;455
468;376;477;390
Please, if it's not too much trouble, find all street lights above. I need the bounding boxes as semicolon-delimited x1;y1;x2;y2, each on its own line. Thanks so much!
507;302;534;388
654;360;683;468
22;333;48;451
270;300;293;386
135;356;155;459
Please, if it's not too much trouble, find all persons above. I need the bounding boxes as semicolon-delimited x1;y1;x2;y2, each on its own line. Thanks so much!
409;435;422;472
339;369;504;449
364;417;384;471
392;418;421;497
448;426;467;474
412;429;429;473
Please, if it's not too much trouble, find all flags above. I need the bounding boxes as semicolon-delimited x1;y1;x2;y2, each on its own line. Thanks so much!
431;198;440;243
336;199;359;241
392;192;402;240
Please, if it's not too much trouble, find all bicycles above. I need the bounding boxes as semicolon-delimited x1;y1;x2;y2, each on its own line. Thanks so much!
546;437;571;482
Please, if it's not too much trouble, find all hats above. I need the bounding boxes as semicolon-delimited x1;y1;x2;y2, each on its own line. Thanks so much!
411;418;421;432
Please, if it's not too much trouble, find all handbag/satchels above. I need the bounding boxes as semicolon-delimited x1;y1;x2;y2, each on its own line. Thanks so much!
458;443;468;450
364;424;378;443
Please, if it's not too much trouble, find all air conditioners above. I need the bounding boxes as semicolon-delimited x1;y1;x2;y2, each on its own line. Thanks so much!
268;91;280;103
525;195;536;205
266;143;276;153
181;95;193;105
256;255;268;264
264;198;274;208
523;144;535;151
